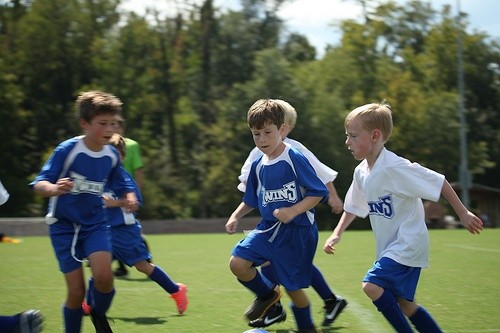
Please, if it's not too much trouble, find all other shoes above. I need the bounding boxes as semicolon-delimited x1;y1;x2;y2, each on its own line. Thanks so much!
115;269;127;276
14;310;42;333
82;304;90;315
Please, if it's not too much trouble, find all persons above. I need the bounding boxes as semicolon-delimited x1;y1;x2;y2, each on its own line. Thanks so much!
225;98;331;333
26;90;122;333
80;132;189;316
324;103;484;333
113;115;151;277
236;100;347;327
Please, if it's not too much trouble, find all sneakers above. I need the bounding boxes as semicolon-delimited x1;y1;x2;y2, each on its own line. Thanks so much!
247;291;280;321
322;298;347;326
250;306;286;327
170;283;188;314
